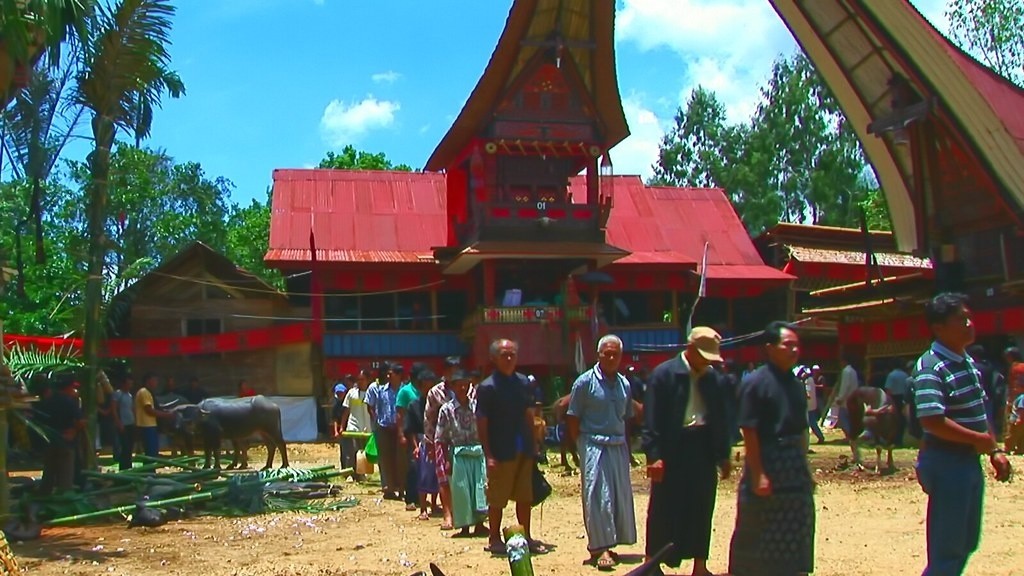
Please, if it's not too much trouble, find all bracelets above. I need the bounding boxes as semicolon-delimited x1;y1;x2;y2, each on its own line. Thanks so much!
991;448;1005;454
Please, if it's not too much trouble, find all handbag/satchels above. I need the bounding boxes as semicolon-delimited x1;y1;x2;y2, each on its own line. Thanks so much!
356;449;375;474
365;433;379;464
822;402;844;429
530;460;552;508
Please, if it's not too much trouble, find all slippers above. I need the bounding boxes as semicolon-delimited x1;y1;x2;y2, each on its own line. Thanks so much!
418;513;430;520
440;522;453;530
484;541;507;554
528;539;548;554
596;557;616;567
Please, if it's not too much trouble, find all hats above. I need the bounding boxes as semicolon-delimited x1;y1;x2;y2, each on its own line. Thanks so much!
688;326;725;361
334;383;347;394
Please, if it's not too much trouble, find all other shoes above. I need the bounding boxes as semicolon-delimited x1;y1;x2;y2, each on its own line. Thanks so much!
406;503;416;511
346;475;353;483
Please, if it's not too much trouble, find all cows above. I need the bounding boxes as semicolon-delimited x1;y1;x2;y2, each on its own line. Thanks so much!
835;385;901;476
156;394;290;471
3;502;41;542
127;501;165;529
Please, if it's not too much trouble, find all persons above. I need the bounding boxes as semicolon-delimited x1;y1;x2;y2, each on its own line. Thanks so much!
728;321;816;576
623;348;1024;455
331;338;548;556
33;373;209;492
911;291;1011;576
238;379;256;397
566;333;639;568
641;325;732;576
